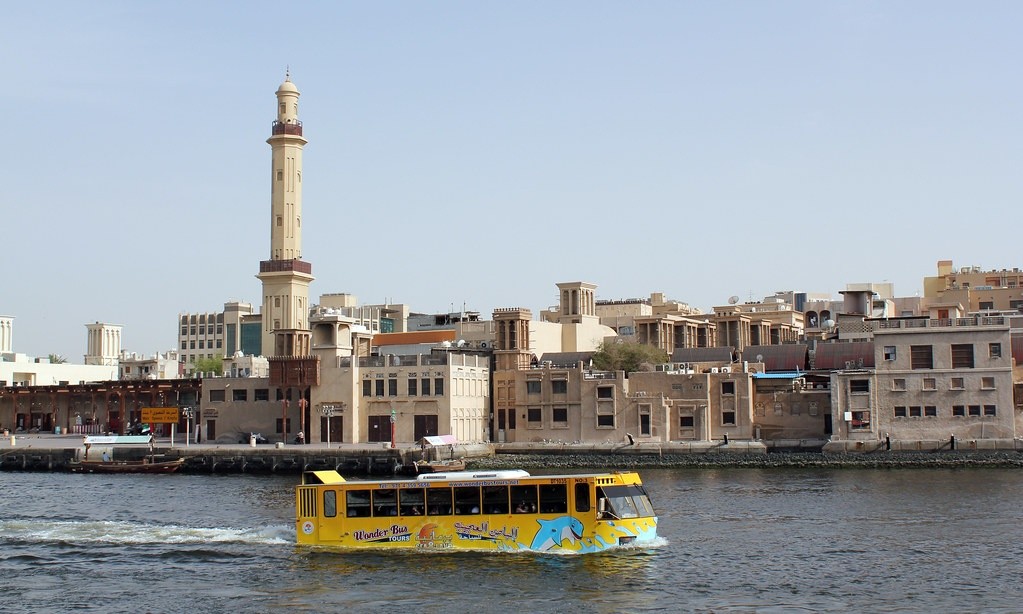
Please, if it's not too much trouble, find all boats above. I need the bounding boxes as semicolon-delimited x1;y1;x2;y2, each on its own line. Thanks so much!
67;435;188;473
391;410;396;448
394;435;466;476
295;469;658;553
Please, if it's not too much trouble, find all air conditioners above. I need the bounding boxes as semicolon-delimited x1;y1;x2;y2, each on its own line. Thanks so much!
663;364;673;371
709;367;719;374
480;340;490;348
721;367;732;373
677;363;686;370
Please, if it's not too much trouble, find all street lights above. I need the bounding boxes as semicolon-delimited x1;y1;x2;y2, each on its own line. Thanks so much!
317;405;335;448
182;407;193;447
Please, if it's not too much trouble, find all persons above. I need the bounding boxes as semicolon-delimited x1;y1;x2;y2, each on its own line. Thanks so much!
516;500;529;514
126;419;130;436
134;416;138;425
411;506;420;515
297;430;304;443
390;506;404;516
103;452;110;461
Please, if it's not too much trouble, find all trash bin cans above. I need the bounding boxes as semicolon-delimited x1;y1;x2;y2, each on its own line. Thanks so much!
62;427;67;435
483;427;490;442
498;429;506;443
55;425;61;435
10;436;16;446
752;423;762;441
250;435;256;448
4;427;9;437
